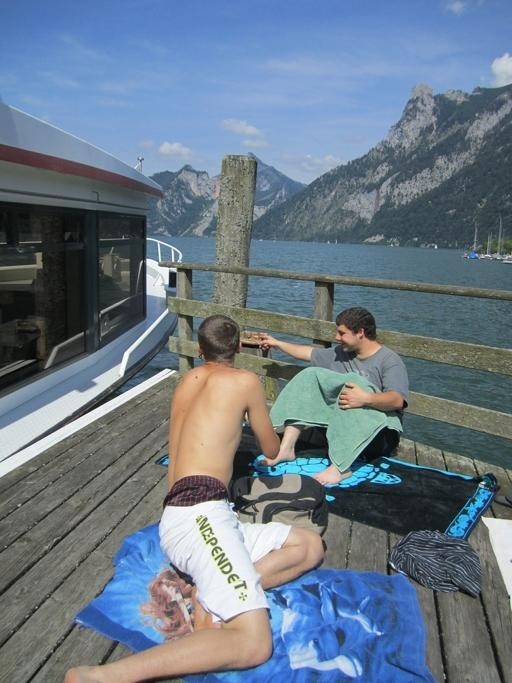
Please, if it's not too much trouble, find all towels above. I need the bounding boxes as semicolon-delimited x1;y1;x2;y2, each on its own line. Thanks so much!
268;366;403;474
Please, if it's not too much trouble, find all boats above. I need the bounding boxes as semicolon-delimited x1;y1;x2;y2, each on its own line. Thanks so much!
0;100;183;463
463;214;512;268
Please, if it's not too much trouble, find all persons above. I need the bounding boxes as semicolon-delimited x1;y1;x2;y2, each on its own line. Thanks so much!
65;315;323;681
257;307;409;486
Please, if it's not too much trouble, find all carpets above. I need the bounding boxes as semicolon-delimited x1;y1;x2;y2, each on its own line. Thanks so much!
75;520;436;682
155;418;500;541
